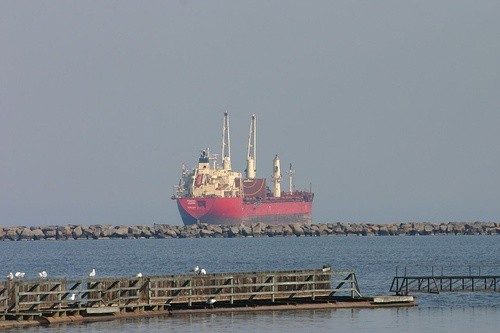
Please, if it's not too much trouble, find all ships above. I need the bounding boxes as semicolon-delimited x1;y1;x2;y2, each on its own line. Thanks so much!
171;113;315;224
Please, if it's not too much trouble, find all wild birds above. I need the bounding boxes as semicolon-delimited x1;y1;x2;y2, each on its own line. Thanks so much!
136;273;142;277
38;271;47;278
198;269;206;275
190;265;199;272
69;293;76;300
14;271;25;279
4;272;14;281
206;297;217;304
88;268;96;277
